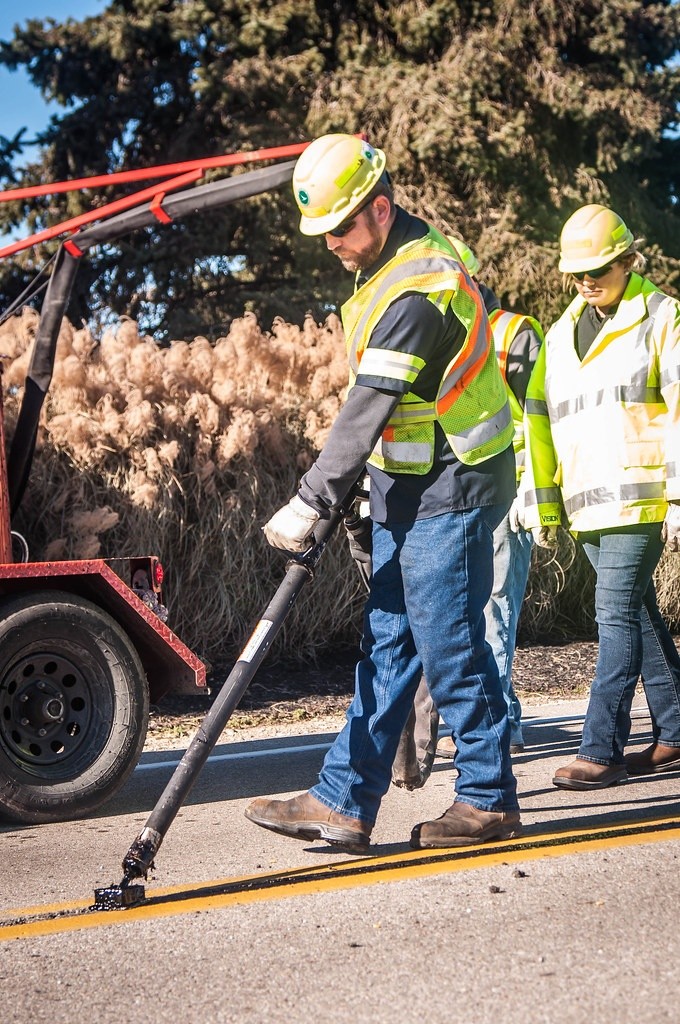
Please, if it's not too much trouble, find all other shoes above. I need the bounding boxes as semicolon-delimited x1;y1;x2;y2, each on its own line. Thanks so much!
436;735;525;760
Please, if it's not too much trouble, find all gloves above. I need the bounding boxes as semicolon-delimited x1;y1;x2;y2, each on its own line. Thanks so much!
508;488;531;533
660;502;680;552
530;523;561;550
262;493;321;553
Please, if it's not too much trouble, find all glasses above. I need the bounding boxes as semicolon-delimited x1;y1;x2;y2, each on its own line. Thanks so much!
323;192;382;239
571;255;624;281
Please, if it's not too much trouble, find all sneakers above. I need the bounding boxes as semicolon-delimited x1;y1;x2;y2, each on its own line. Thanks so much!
625;740;680;776
552;758;629;790
410;799;522;849
244;789;375;854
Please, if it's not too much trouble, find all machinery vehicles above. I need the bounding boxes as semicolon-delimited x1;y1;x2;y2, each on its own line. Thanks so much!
1;125;373;832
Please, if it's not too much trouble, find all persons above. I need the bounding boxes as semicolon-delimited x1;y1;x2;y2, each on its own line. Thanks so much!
246;134;528;849
434;233;546;763
523;202;680;791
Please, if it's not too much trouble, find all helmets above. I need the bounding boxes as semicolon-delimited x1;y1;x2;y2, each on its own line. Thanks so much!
558;204;635;272
447;235;482;278
293;134;386;237
